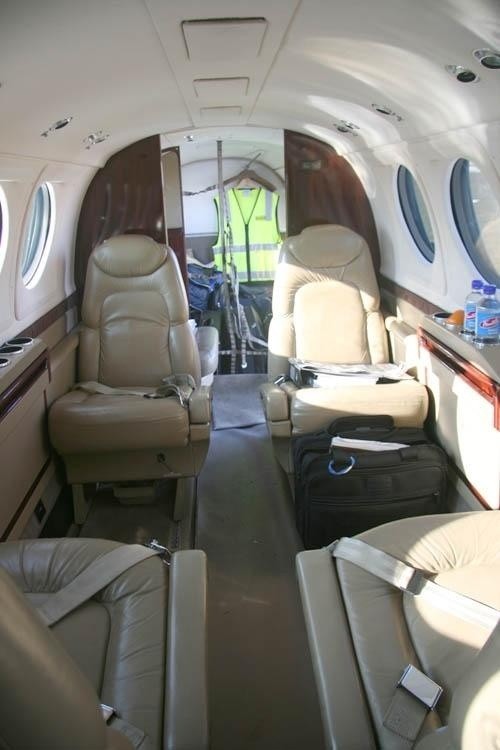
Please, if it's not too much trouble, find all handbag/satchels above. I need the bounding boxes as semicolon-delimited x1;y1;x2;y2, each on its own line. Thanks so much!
293;415;450;551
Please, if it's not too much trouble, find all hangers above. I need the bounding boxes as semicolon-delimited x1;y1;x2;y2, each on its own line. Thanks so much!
234;178;257;190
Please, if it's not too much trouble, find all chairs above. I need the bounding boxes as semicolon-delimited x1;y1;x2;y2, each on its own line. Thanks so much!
296;510;500;750
258;224;430;504
0;537;209;750
46;234;219;524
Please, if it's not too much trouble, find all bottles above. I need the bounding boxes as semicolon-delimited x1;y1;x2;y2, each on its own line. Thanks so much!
463;279;484;330
475;284;500;341
